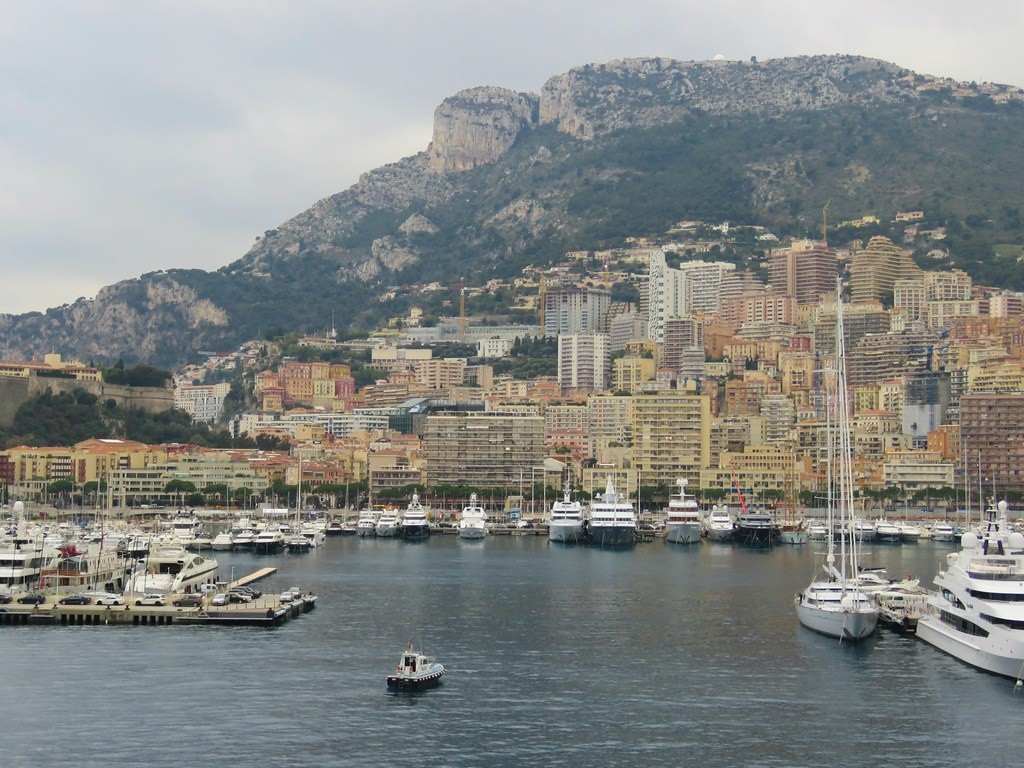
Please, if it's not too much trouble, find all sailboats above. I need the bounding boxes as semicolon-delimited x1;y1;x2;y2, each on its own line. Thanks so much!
0;478;151;592
488;469;550;534
641;276;1023;682
155;479;327;552
328;474;358;534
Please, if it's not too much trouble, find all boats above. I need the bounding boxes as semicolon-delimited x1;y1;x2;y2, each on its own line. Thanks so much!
549;466;584;543
359;487;487;539
386;643;448;692
124;533;219;594
587;463;637;548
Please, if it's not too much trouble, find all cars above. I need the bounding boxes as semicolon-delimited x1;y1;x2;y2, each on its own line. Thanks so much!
212;593;230;606
94;595;125;606
133;593;166;606
172;595;203;607
279;592;295;602
16;593;45;605
0;593;13;604
289;587;301;598
59;594;91;605
223;586;261;604
